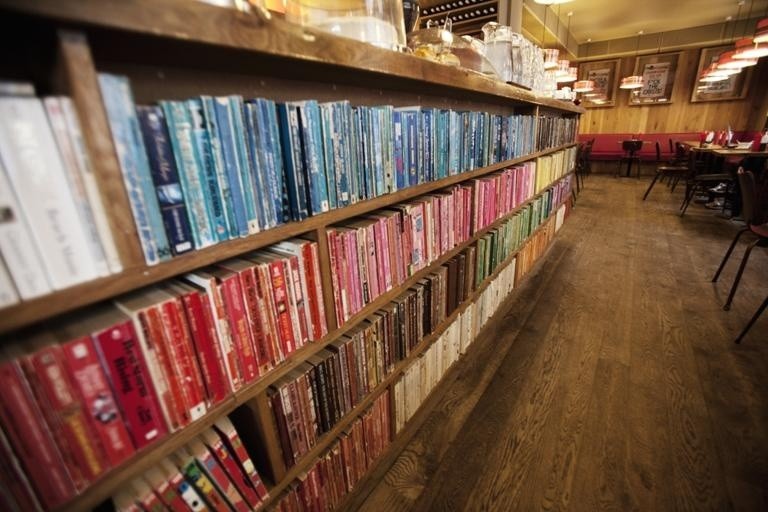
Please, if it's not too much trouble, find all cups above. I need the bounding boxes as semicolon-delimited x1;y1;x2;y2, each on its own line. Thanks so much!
700;131;709;147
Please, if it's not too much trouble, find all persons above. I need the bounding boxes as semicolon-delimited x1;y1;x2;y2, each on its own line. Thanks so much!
714;144;767;216
705;181;734;209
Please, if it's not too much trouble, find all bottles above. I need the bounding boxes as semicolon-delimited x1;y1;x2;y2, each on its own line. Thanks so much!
752;131;767;152
713;128;726;145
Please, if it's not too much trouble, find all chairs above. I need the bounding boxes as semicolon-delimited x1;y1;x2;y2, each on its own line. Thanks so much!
577;137;768;344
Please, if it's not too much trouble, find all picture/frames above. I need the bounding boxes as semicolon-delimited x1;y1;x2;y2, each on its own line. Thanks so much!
576;59;620;109
689;47;752;104
626;51;685;105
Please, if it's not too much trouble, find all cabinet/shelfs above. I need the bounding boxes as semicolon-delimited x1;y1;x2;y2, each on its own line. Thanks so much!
0;0;583;512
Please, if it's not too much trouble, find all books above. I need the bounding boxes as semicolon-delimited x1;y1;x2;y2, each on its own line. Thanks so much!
1;75;579;510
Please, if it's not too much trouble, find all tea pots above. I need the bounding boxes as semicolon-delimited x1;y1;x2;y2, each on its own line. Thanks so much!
482;20;545;93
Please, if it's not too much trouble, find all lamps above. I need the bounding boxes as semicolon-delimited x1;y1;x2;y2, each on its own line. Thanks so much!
697;0;768;83
618;29;645;90
538;0;612;105
